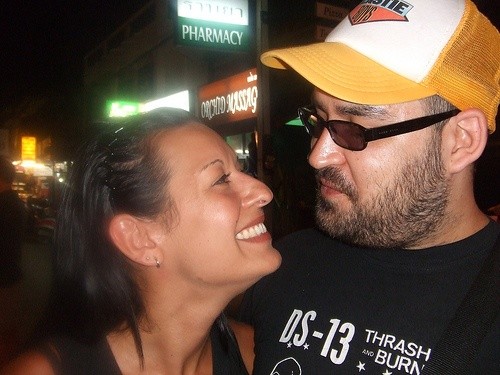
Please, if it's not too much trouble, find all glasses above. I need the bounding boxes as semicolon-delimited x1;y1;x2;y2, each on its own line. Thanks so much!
297;105;460;151
96;109;152;189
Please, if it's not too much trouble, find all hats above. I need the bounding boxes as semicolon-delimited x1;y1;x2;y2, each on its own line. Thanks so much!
260;0;500;134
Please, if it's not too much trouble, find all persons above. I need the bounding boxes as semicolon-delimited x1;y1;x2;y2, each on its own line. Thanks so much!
0;106;283;375
0;157;29;365
229;0;500;375
